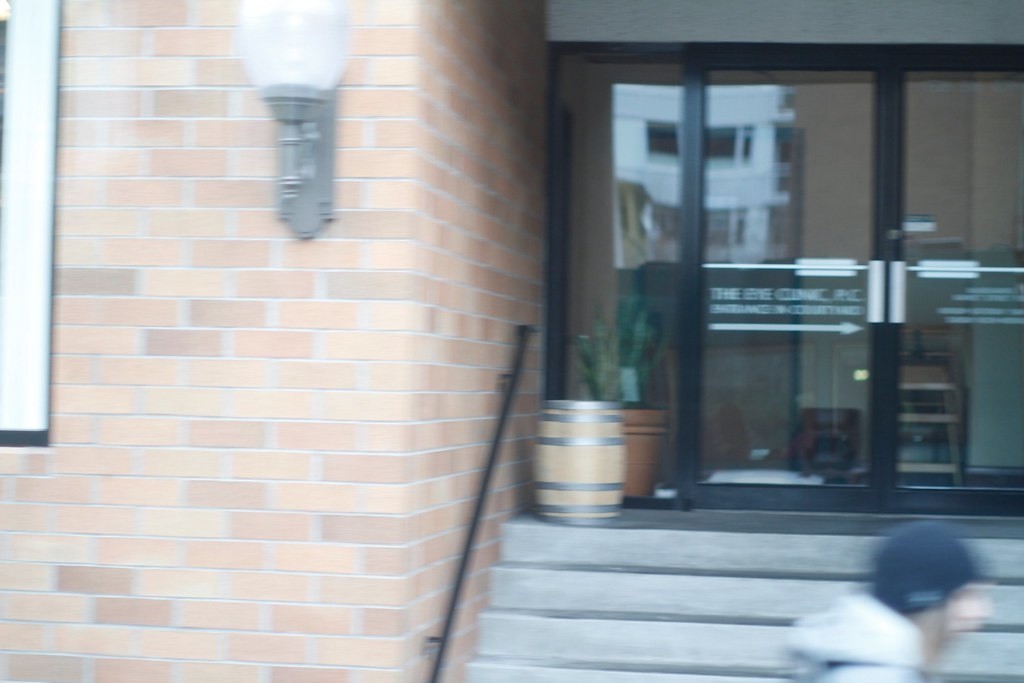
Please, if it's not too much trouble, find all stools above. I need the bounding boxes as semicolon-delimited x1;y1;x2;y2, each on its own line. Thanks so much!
794;407;861;477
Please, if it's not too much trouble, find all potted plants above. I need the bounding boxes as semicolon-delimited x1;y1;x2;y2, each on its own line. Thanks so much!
577;289;679;496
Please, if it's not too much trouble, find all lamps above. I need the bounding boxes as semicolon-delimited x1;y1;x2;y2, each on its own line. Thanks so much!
237;1;354;240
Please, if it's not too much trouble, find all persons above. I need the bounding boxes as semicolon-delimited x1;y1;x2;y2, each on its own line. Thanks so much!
789;521;981;683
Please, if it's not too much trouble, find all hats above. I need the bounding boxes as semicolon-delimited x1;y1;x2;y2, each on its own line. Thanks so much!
867;523;981;617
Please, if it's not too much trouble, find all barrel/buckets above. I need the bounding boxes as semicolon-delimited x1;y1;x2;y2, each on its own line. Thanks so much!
532;396;629;526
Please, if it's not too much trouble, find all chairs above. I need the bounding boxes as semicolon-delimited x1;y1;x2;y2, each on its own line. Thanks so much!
895;323;964;489
